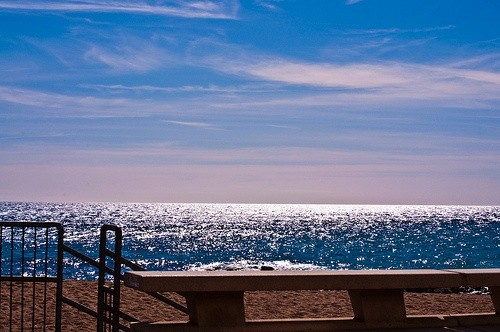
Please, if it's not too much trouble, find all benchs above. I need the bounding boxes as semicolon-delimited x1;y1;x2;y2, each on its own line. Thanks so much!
444;269;500;331
123;268;462;332
